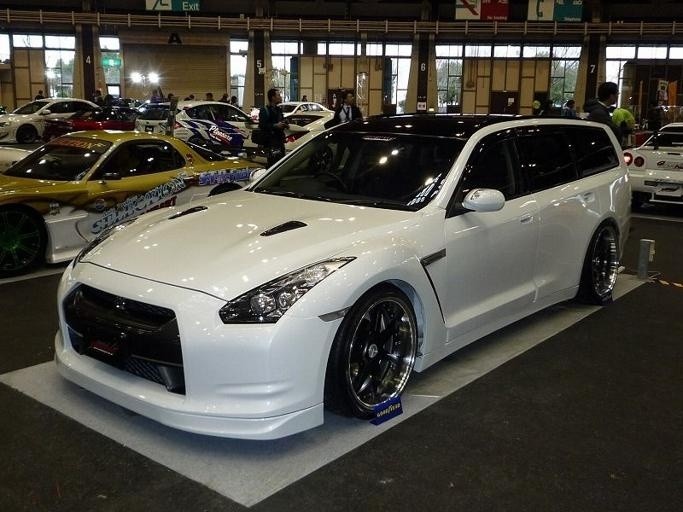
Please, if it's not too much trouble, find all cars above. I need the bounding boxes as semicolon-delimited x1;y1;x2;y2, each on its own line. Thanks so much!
52;113;634;442
0;89;335;163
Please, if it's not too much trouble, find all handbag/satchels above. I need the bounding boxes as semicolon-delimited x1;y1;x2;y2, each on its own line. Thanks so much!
252;128;273;145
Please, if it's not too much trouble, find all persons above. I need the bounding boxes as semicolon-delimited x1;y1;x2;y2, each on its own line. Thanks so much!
259;88;290;169
35;90;45;100
333;92;364;175
91;89;106;107
535;80;637;149
299;94;310;102
146;86;244;108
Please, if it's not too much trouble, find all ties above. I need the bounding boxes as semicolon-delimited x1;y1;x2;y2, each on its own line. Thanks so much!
345;107;349;122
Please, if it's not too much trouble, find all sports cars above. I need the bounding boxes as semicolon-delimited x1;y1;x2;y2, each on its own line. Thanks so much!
620;120;682;205
0;132;265;274
